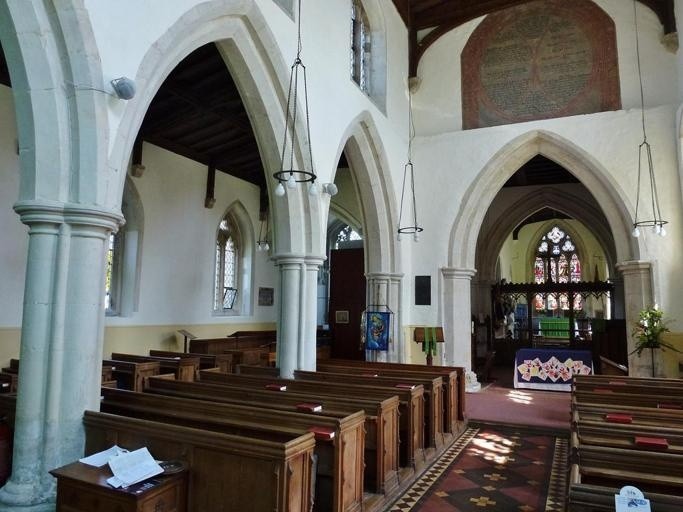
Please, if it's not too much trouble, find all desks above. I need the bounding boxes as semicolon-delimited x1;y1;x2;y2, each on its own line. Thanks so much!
48;453;191;512
513;348;596;393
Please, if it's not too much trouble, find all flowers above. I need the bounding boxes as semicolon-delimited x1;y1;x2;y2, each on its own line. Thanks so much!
627;304;681;358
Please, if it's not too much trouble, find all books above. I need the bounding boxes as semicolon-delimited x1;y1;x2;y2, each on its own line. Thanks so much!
78;444;164;489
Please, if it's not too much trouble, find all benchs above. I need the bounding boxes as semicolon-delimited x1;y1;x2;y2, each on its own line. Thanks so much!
199;370;426;475
572;395;683;418
571;409;683;453
0;394;17;430
82;410;317;512
572;385;683;406
112;353;200;382
568;464;683;512
571;377;683;395
101;380;367;512
103;359;160;393
572;373;683;382
150;350;232;373
570;430;683;486
239;365;445;455
271;360;460;439
0;358;19;393
142;373;402;503
316;358;466;426
101;365;117;388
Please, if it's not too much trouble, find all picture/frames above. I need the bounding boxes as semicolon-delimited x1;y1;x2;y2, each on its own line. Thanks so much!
258;287;274;306
223;289;238;309
335;311;349;323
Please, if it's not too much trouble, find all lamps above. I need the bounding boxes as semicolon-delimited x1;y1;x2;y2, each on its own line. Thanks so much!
326;182;339;196
272;0;318;198
396;79;424;244
111;76;137;101
255;187;271;252
630;0;669;240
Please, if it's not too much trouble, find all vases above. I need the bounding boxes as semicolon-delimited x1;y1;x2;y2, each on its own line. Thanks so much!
643;343;660;348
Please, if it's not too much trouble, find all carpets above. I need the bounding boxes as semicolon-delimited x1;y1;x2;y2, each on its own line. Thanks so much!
378;423;569;512
